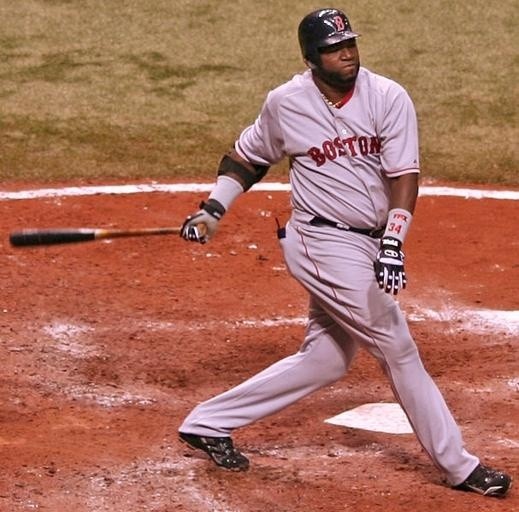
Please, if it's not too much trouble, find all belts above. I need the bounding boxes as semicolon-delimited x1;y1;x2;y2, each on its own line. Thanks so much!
314;215;385;240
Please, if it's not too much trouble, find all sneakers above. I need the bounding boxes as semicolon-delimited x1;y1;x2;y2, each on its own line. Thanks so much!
178;430;249;472
451;463;513;499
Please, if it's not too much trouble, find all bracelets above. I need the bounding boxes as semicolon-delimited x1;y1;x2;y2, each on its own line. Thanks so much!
382;208;414;244
208;174;244;214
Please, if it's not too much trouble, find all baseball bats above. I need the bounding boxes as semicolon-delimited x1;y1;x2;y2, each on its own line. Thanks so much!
9;222;207;245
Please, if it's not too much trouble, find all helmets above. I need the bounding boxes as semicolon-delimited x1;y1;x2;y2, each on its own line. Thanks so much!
297;8;362;57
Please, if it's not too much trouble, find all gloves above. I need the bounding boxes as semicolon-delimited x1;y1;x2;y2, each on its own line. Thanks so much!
180;199;227;244
374;235;408;295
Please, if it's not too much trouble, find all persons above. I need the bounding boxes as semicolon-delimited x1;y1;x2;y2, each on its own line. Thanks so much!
179;8;513;498
